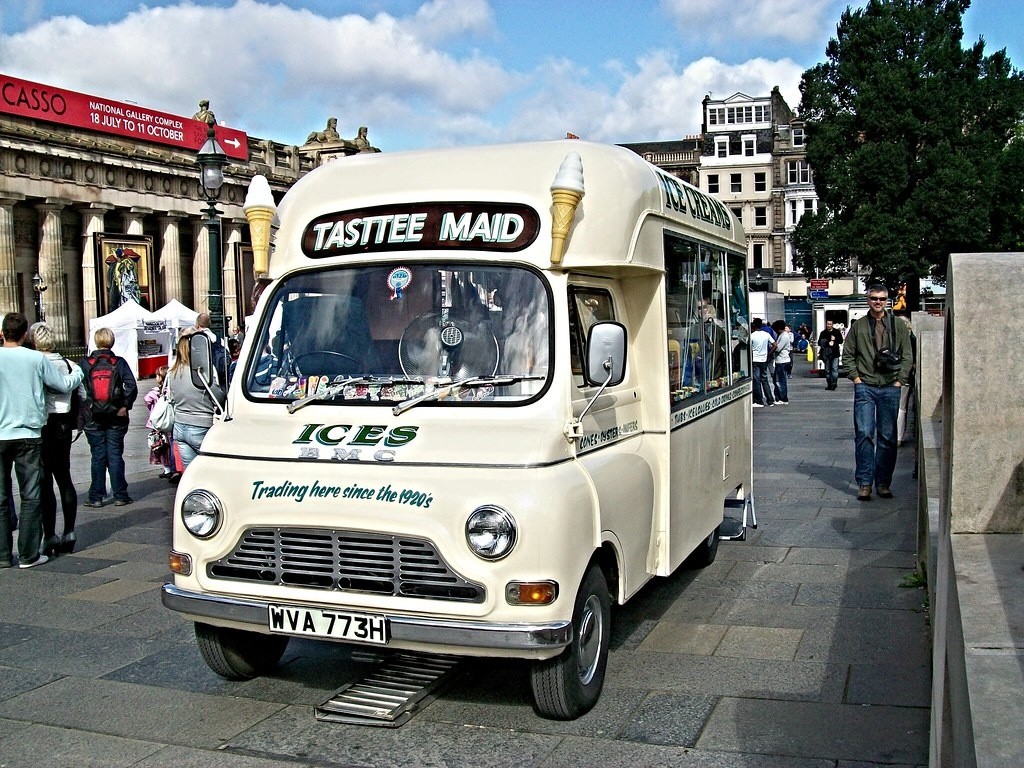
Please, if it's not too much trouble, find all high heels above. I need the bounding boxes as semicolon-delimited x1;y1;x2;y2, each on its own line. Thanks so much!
58;532;77;554
39;535;60;557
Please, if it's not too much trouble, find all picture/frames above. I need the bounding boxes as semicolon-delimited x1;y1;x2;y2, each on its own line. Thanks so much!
92;231;156;318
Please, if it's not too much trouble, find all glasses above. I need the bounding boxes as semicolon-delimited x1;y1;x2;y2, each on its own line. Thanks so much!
869;296;887;302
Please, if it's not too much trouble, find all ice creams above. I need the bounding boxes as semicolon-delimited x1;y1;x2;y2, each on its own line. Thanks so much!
549;150;585;263
243;174;277;274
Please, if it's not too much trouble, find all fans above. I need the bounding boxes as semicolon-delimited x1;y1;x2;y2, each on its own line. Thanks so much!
398;306;500;377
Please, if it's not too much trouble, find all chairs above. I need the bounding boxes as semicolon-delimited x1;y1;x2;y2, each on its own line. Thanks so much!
283;295;385;375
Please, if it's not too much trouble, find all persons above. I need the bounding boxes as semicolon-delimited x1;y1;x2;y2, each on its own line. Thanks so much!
193;100;217;125
682;299;726;388
897;316;917;446
324;118;339;140
750;318;794;408
842;285;913;499
31;321;87;556
355;127;370;149
818;320;843;390
81;328;138;507
144;314;282;483
797;334;809;354
0;312;84;568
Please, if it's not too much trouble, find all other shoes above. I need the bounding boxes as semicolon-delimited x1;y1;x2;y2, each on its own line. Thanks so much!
83;499;102;506
857;485;872;500
753;403;764;408
115;497;133;506
18;555;49;568
168;472;181;484
0;560;12;567
877;484;892;497
159;471;172;478
773;400;789;406
766;403;774;407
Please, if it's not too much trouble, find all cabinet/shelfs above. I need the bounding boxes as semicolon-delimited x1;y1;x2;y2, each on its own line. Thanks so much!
138;354;168;379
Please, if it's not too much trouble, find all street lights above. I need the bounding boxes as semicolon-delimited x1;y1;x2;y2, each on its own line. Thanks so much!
194;117;235;392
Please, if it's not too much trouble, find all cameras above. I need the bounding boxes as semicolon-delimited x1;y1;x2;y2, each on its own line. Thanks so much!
875;348;900;369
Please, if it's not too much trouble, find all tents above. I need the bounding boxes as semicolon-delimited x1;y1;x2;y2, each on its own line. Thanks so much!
88;300;199;379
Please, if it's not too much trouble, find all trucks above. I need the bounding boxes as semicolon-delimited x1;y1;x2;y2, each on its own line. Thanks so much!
749;291;786;329
808;302;893;373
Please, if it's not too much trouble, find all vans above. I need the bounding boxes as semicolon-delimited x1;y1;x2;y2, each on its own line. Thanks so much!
165;138;764;715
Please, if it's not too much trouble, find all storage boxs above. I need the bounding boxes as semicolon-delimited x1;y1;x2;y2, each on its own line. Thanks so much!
139;345;160;356
145;340;156;346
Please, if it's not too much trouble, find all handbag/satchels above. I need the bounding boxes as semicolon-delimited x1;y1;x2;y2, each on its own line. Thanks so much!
149;368;175;433
62;357;93;430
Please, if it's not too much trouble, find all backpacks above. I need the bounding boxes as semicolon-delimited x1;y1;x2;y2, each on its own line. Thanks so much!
83;354;122;419
